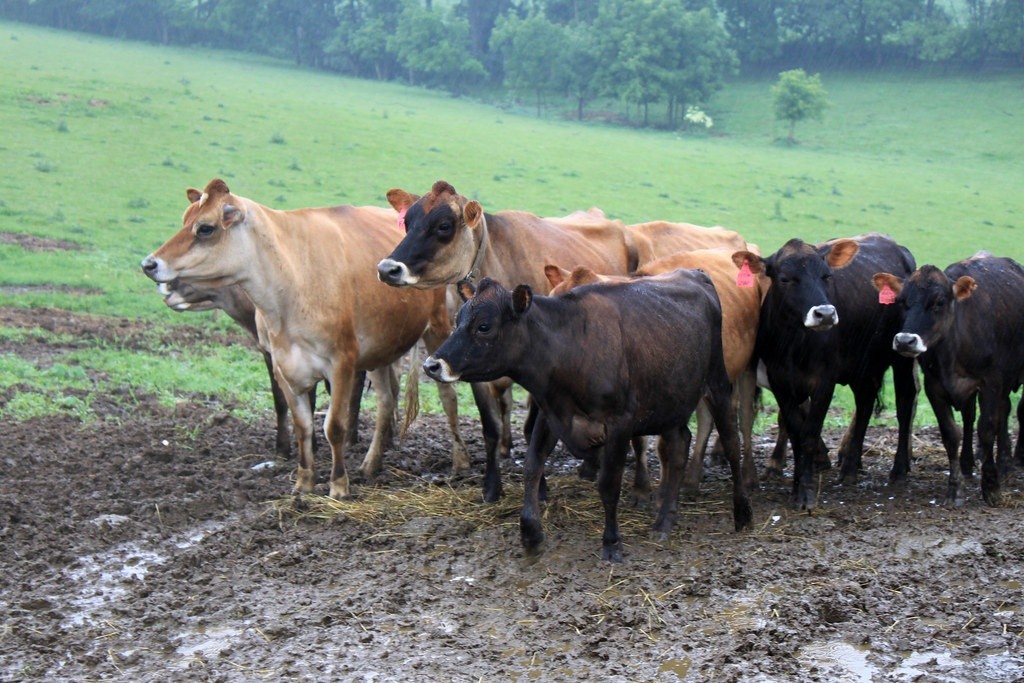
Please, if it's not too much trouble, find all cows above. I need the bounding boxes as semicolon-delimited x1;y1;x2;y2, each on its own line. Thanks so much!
140;178;1024;562
